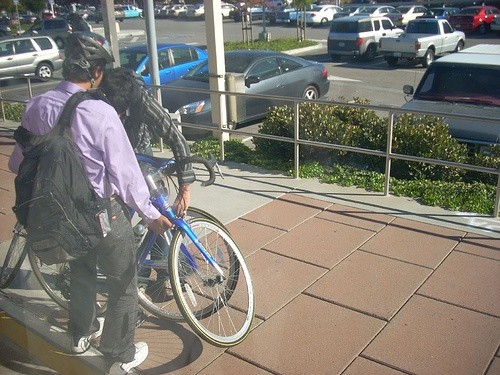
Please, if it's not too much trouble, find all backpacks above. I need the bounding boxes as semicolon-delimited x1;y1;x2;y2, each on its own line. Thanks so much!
13;90;110;265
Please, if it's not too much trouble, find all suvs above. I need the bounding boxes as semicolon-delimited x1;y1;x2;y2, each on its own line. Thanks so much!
327;17;405;62
20;19;72;49
398;44;500;156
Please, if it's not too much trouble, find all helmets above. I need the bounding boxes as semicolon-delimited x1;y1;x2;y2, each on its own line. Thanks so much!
65;31;115;68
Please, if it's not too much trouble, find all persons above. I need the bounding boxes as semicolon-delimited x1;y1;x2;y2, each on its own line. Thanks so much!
7;31;172;375
100;66;195;301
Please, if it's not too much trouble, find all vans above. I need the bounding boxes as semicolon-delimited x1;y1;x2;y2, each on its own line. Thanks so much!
0;36;64;82
94;4;124;23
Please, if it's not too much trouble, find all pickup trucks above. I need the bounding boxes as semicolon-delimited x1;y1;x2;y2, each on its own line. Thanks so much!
379;19;465;67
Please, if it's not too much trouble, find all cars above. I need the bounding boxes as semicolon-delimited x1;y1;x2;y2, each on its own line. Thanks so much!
161;51;330;135
354;5;401;17
385;4;434;30
119;42;209;93
234;5;268;21
290;5;343;27
449;6;500;34
416;7;460;22
122;5;142;18
187;4;205;19
333;6;362;18
168;4;186;18
268;1;317;23
222;3;238;19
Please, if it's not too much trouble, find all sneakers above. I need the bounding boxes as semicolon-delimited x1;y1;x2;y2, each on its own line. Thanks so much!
106;342;148;375
71;317;105;354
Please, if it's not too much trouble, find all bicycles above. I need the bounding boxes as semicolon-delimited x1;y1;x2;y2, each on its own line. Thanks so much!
0;205;239;321
29;156;255;348
